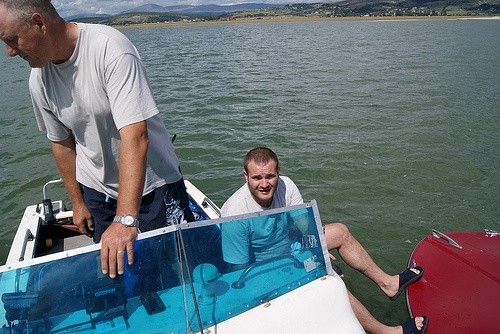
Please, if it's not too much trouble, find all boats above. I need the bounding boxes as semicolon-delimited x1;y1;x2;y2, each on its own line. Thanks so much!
406;229;500;334
0;175;366;334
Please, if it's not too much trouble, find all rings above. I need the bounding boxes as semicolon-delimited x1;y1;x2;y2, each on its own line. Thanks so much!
117;251;125;254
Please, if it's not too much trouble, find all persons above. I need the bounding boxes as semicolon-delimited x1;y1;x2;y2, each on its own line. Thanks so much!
220;147;429;334
0;0;195;299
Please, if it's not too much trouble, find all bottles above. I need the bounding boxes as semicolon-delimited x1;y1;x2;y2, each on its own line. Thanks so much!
42;199;54;222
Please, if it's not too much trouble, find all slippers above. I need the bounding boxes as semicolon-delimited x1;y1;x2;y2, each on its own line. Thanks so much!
389;266;424;301
397;315;429;334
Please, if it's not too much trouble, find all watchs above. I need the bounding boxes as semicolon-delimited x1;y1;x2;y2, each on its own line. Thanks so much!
113;214;139;228
